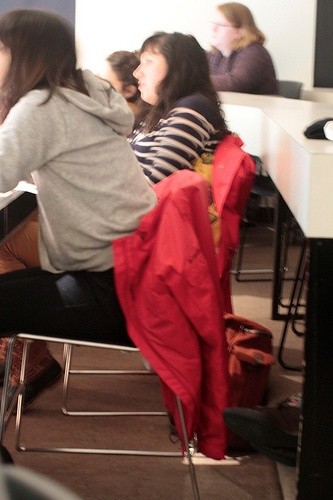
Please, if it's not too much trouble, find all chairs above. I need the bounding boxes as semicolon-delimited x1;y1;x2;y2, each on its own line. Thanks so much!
0;77;303;500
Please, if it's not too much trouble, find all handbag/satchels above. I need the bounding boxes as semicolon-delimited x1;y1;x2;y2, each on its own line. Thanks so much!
220;311;276;408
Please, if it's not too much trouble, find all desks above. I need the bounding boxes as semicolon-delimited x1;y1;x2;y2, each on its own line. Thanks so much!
214;91;333;500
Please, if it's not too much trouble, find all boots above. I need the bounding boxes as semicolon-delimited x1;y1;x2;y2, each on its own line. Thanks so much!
0;336;62;413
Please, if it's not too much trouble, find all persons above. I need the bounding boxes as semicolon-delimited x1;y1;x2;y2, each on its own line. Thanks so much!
0;32;228;417
204;3;277;98
